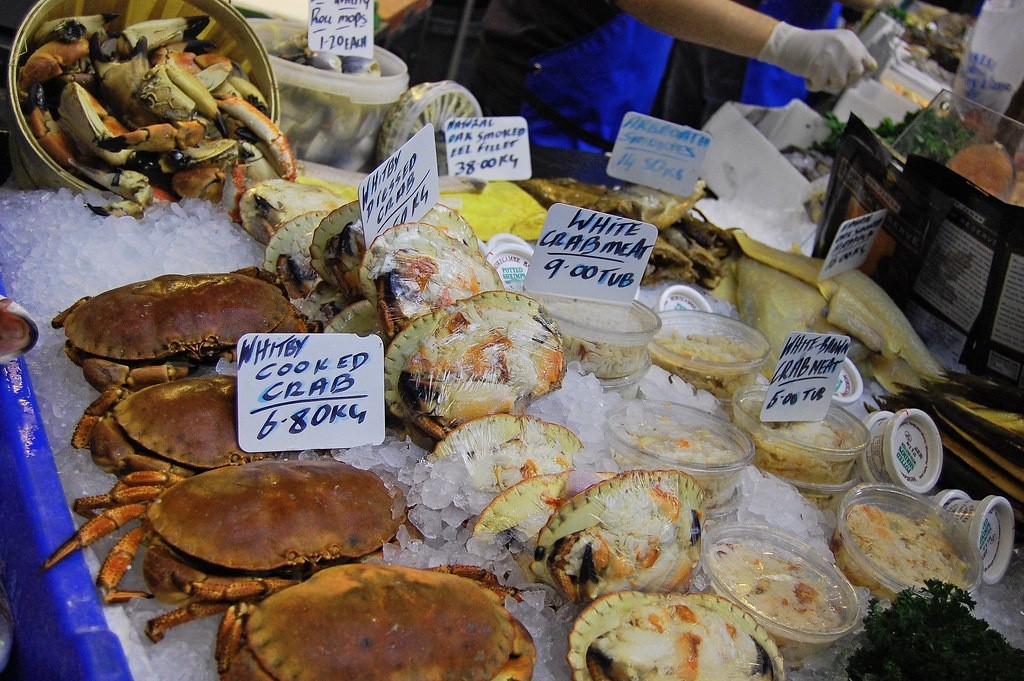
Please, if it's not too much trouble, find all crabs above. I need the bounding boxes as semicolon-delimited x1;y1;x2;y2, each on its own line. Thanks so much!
14;0;977;681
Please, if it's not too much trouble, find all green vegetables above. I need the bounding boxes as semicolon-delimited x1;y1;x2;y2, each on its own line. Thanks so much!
843;577;1024;681
823;98;974;167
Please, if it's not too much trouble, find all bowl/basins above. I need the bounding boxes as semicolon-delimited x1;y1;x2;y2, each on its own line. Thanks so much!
541;294;660;389
645;310;771;412
693;524;862;667
830;481;984;617
605;397;754;518
730;390;871;544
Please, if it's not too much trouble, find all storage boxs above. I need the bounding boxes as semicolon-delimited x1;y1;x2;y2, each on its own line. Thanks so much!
701;96;833;208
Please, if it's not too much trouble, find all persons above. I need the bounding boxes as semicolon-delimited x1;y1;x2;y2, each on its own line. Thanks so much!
458;0;876;186
665;0;880;133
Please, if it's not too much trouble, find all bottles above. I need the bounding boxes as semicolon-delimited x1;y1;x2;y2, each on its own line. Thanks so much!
951;0;1022;143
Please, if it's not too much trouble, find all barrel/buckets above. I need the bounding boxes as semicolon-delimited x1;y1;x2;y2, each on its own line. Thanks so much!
7;1;281;194
243;15;410;174
